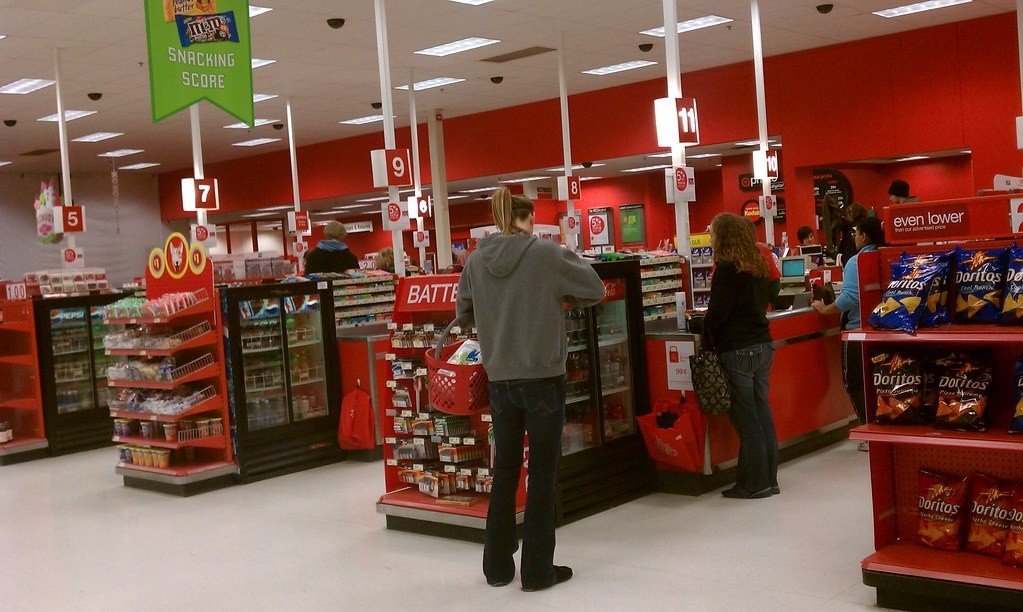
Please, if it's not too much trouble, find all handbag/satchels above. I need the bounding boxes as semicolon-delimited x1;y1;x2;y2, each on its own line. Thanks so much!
688;343;731;415
636;400;702;473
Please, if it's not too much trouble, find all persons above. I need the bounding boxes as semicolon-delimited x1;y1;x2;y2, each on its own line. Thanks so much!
887;179;920;204
455;187;605;591
831;217;858;268
846;203;868;222
375;248;410;277
811;215;889;450
701;212;780;500
709;216;781;312
793;226;826;266
303;221;359;274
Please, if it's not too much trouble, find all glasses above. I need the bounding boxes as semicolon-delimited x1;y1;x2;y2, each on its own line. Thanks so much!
851;233;863;238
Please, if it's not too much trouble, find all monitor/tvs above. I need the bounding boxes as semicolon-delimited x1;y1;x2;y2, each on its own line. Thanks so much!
779;255;817;287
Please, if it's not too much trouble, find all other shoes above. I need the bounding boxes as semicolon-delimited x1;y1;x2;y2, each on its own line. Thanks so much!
768;477;781;496
858;441;869;452
520;565;573;592
484;570;514;587
721;482;771;499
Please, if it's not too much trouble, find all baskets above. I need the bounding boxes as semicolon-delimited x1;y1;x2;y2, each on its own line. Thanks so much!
424;318;490;417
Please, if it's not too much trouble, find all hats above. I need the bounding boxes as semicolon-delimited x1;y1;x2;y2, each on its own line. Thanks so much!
888;179;910;198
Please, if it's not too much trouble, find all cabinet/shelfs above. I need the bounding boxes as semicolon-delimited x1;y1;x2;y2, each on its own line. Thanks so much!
103;267;399;498
0;289;146;465
378;245;714;544
840;239;1022;612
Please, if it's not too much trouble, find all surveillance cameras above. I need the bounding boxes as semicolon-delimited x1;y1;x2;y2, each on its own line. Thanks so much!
371;102;382;109
639;44;653;52
87;93;102;100
3;120;17;127
273;124;284;130
816;4;834;14
490;77;503;84
326;18;345;29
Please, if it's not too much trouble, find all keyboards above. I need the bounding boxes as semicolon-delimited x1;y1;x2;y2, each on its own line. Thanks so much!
780;287;806;295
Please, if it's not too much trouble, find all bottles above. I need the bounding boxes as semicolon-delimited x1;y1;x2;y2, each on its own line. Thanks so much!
561;306;628;454
241;314;317;429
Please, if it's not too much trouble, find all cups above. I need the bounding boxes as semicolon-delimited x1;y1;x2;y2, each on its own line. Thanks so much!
114;417;223;469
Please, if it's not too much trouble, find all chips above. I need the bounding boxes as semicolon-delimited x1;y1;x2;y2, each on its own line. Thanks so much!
874;392;1023;422
872;290;1023;319
918;522;1023;557
